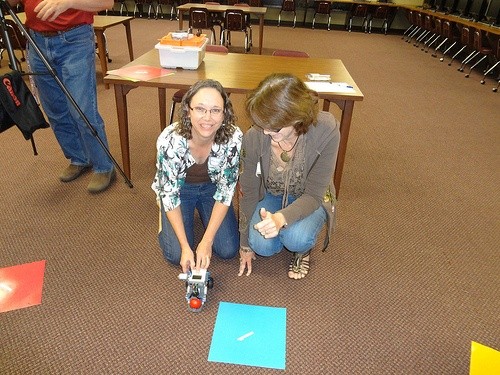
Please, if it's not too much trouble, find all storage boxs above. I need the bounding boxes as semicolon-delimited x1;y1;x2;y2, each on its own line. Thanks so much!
155;37;209;70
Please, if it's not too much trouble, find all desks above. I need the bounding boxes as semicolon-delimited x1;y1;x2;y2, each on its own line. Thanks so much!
103;49;365;200
176;3;267;55
4;11;134;90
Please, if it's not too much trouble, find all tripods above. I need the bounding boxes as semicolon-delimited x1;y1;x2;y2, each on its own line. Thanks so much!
0;0;133;189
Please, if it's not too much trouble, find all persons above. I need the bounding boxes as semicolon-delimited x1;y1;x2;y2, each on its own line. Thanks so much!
151;79;243;273
238;72;340;280
4;0;114;192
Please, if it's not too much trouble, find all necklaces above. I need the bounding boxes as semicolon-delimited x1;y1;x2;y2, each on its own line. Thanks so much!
278;135;299;162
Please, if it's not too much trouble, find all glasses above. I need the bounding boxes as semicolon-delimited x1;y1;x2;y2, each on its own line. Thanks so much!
254;123;283;133
188;102;225;116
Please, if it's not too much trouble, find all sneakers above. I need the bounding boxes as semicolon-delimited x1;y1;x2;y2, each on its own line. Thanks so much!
87;167;116;193
59;164;93;182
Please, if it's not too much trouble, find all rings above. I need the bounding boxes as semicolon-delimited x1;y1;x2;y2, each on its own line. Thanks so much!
265;231;267;234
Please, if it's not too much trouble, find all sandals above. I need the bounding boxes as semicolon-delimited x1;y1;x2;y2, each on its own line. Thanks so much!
288;250;310;280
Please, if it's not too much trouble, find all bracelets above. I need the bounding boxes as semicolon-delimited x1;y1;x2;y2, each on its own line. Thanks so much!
240;247;251;252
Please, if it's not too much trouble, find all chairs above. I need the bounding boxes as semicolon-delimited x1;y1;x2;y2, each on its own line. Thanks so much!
0;18;26;69
312;1;331;31
168;46;229;124
105;0;263;54
277;0;296;29
367;6;388;35
401;12;500;92
346;4;368;33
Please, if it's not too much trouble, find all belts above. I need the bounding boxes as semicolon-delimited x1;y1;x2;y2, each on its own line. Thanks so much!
36;24;81;37
